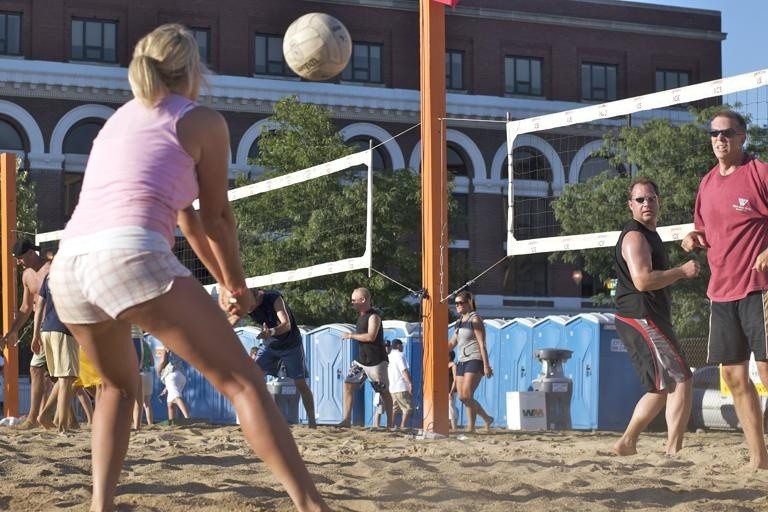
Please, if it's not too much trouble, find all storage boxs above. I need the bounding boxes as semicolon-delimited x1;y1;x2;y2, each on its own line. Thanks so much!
505;391;548;432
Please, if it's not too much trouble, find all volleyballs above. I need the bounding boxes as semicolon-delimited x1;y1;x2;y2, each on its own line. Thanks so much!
283;12;352;81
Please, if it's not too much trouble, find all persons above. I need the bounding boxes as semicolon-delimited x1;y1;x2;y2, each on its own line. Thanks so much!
682;111;768;472
333;287;393;429
387;339;415;430
0;238;189;429
47;22;329;511
248;287;317;428
449;351;457;430
613;177;701;457
448;291;494;433
250;347;258;360
373;340;390;428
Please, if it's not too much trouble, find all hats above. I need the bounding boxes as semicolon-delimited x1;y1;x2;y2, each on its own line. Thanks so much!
13;240;40;256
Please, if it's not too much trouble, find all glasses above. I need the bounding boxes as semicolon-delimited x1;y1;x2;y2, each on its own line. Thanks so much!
636;197;656;202
710;128;736;136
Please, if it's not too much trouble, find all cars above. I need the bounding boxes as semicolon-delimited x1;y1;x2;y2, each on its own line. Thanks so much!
687;364;767;433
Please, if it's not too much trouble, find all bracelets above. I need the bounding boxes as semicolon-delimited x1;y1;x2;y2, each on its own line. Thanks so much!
270;329;275;337
224;286;248;303
